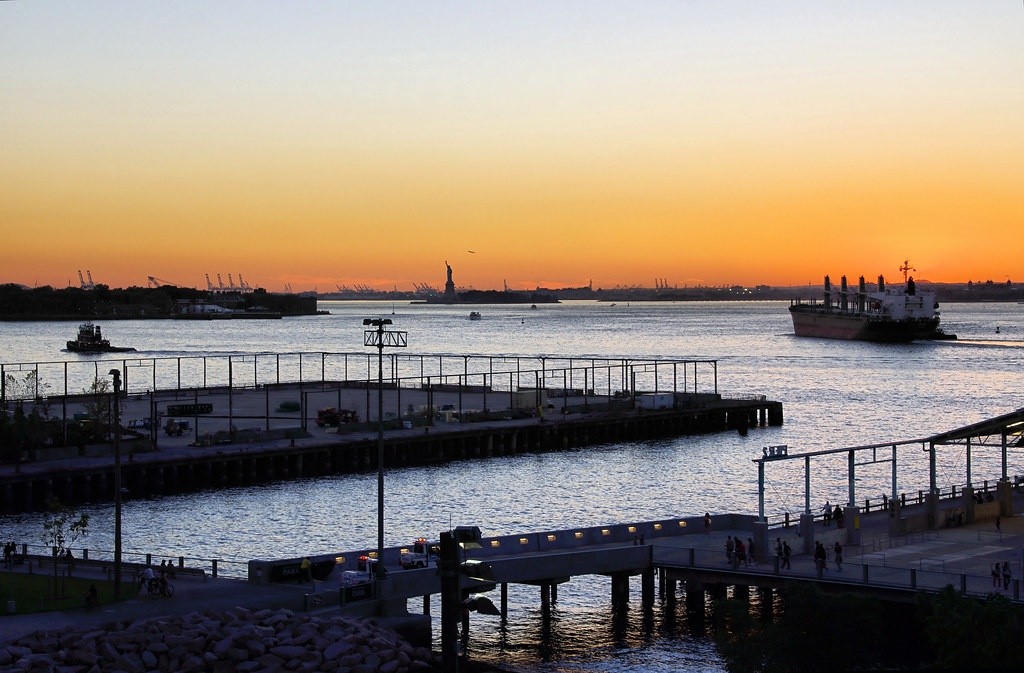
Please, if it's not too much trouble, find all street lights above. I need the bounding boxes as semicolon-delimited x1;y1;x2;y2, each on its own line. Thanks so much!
108;368;122;602
363;316;394;583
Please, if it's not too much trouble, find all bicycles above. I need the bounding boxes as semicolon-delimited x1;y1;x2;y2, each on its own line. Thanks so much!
148;578;174;600
136;573;153;590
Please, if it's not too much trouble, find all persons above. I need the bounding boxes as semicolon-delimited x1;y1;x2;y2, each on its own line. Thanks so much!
88;584;101;611
775;538;785;562
3;542;16;568
161;559;177;580
704;512;711;533
138;566;171;598
974;490;994;504
834;542;842;571
747;538;759;565
781;538;791;570
634;534;645;546
301;556;312;582
814;541;828;572
832;501;843;528
724;536;747;569
820;501;832;526
1002;561;1012;592
59;549;76;571
993;563;1001;590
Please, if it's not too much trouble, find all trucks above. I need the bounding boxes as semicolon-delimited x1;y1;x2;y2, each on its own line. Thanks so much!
400;538;440;569
339;556;380;588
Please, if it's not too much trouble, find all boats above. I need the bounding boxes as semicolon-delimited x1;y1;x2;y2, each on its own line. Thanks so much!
66;322;103;354
787;272;940;343
